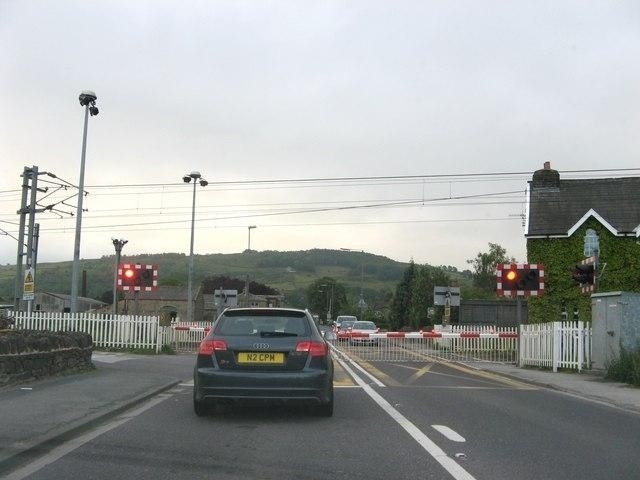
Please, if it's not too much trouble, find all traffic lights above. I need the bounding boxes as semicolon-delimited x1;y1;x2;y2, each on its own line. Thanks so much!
496;263;544;297
571;264;594;285
117;263;158;292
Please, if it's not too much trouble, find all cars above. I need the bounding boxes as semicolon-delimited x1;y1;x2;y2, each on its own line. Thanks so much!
193;307;334;418
348;320;381;347
333;315;357;334
336;321;354;342
